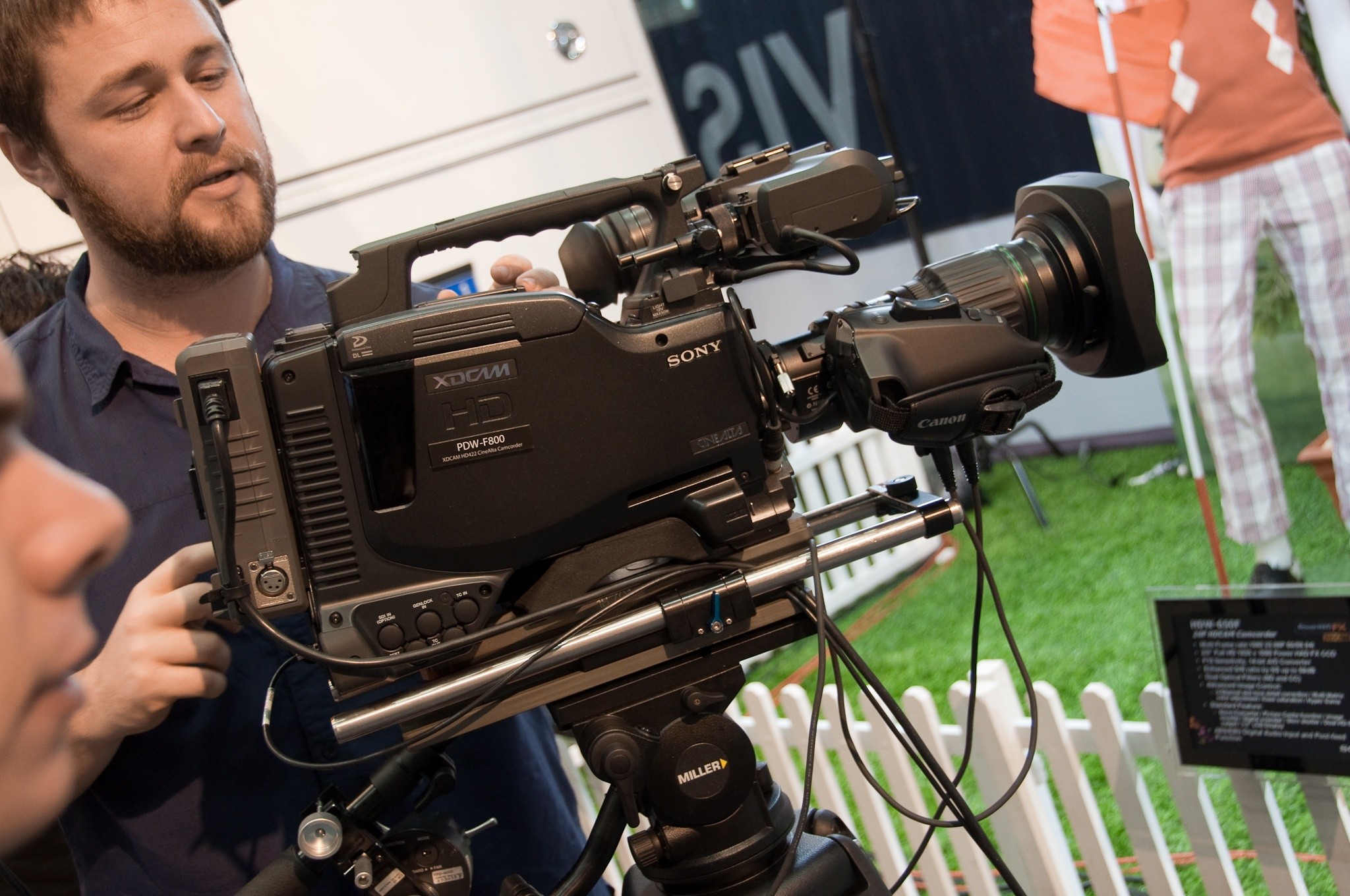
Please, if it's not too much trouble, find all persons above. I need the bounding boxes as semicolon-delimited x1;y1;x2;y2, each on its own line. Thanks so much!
0;337;134;847
0;0;616;896
1032;0;1350;590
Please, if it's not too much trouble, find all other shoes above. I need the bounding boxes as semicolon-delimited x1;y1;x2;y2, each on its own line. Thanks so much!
1246;561;1306;589
